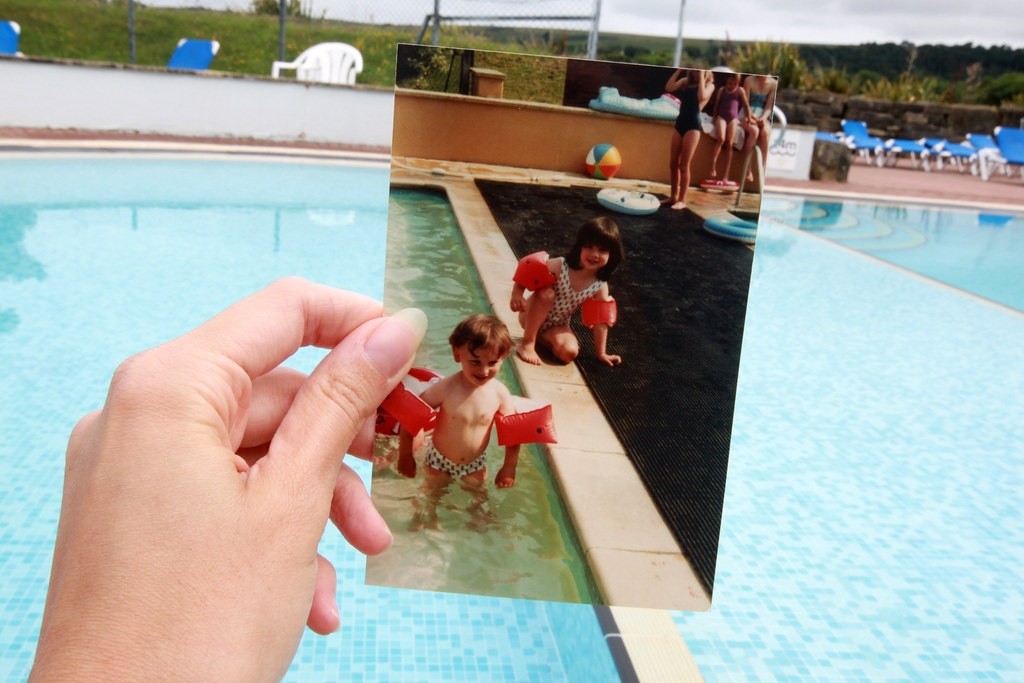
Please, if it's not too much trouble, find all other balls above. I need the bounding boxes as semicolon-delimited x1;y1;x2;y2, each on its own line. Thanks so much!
585;142;622;181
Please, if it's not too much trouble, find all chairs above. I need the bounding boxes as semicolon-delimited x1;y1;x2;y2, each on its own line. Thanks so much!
0;20;26;56
272;43;362;90
168;37;222;69
817;120;1024;182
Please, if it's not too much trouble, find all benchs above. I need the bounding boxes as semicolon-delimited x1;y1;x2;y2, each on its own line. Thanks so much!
470;67;506;100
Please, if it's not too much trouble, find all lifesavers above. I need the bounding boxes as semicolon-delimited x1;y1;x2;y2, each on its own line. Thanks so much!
374;365;560;449
512;250;619;329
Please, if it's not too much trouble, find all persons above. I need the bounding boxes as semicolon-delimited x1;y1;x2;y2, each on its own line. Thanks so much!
710;74;778;206
397;313;521;487
25;277;428;683
510;217;621;366
660;69;715;209
